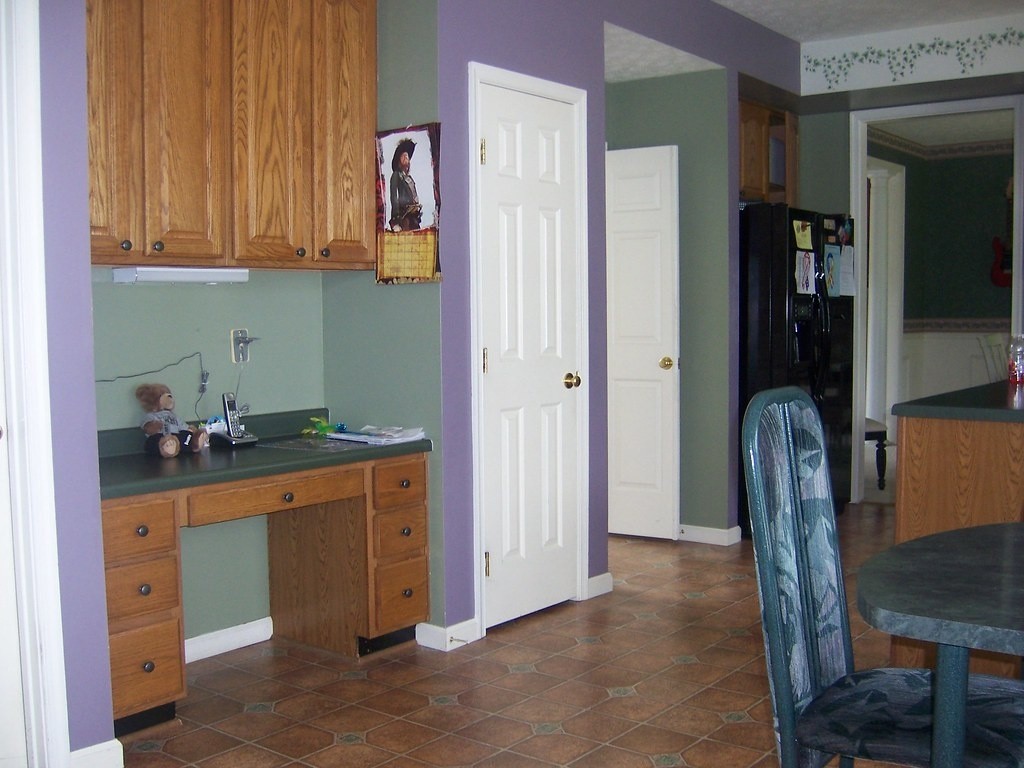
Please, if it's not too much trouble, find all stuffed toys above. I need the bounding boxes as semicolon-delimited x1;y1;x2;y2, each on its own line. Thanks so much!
133;382;207;458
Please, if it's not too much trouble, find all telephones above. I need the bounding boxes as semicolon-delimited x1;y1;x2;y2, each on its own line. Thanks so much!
208;392;259;447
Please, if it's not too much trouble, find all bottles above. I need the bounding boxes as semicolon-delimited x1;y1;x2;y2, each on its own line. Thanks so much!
1011;335;1024;383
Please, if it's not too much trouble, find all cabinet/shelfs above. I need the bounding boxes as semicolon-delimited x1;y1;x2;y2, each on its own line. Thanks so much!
86;0;377;270
738;94;800;208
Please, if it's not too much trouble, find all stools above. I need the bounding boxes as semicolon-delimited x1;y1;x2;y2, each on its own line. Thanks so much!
865;418;887;490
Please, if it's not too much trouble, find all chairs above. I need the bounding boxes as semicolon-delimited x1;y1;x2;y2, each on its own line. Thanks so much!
741;386;1023;768
976;333;1009;384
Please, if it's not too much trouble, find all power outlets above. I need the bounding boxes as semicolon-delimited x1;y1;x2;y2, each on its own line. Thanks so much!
230;328;250;363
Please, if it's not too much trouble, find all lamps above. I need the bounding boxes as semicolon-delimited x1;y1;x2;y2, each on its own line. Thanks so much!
112;267;248;285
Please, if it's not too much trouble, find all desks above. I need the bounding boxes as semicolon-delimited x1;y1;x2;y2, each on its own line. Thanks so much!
100;429;433;739
889;377;1024;681
857;523;1024;768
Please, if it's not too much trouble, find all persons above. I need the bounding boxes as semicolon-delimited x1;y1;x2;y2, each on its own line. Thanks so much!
389;137;423;232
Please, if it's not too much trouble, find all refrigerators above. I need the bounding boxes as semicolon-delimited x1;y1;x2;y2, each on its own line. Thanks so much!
740;202;854;536
